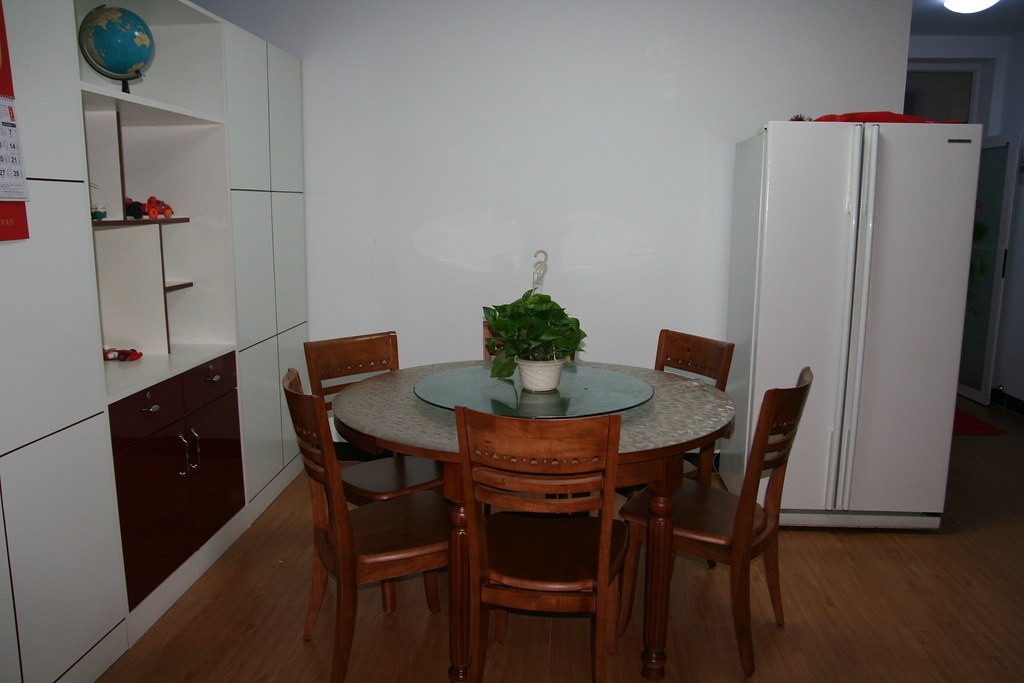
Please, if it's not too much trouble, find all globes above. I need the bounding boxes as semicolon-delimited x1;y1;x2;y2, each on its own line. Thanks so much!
78;4;155;94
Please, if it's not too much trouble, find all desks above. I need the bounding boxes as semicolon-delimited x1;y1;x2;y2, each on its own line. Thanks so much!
331;360;737;683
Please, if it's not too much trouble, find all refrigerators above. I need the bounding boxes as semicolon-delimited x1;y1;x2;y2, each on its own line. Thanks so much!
718;121;984;528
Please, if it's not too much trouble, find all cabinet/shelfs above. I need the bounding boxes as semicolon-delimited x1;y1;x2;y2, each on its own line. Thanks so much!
0;0;310;683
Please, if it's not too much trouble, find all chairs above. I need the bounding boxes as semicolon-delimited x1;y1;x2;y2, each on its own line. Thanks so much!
455;405;628;683
483;321;735;568
282;368;450;683
304;331;444;615
616;366;814;675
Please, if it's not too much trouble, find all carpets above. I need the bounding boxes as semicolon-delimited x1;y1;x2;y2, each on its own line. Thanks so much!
951;406;1006;435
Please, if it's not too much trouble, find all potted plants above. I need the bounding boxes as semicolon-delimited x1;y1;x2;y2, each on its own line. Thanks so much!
482;249;588;378
